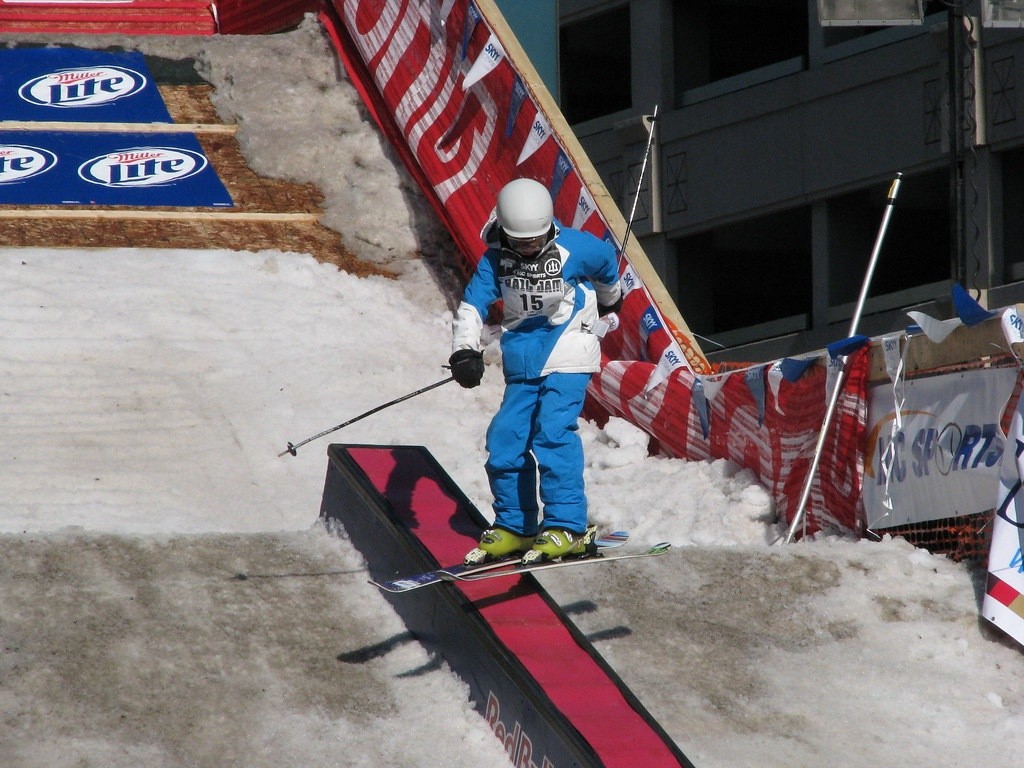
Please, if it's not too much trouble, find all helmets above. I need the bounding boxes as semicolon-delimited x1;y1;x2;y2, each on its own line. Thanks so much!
496;178;555;238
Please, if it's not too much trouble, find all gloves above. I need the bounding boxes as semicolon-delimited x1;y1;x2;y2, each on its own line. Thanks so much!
449;349;486;389
598;290;624;316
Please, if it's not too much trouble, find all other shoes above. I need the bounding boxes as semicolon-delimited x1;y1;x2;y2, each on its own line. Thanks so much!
478;526;540;563
527;524;600;562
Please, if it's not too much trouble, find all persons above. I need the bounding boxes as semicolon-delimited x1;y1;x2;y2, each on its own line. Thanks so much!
450;177;621;564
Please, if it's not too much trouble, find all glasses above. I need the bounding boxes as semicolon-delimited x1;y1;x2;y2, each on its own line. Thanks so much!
504;235;546;250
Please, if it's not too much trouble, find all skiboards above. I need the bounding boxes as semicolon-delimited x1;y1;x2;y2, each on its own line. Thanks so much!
366;525;630;596
432;539;674;584
